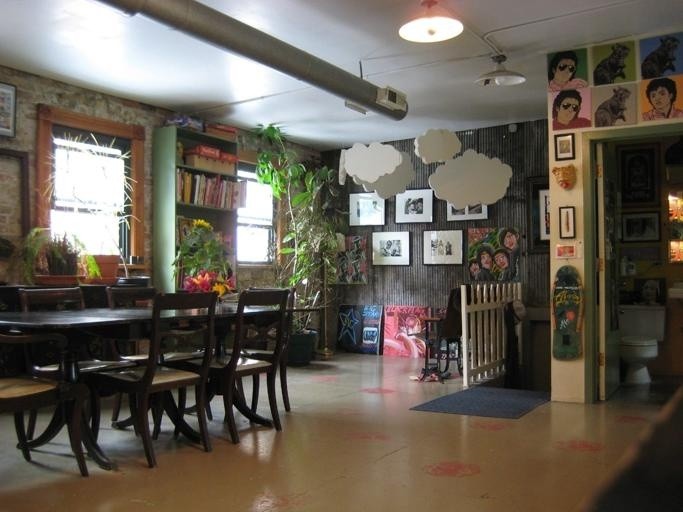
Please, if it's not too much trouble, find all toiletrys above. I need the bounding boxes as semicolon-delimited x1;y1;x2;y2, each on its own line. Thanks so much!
626;258;637;276
605;239;611;260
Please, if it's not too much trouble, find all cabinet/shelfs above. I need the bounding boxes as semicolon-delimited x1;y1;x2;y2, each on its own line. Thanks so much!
0;284;155;398
152;126;240;351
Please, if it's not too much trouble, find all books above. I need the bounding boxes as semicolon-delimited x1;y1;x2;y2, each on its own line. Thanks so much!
176;168;248;209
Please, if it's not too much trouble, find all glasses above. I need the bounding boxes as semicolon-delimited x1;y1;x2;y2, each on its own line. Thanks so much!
556;63;576;73
560;102;579;112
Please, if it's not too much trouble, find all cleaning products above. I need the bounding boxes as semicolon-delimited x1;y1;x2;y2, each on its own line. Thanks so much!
620;256;627;276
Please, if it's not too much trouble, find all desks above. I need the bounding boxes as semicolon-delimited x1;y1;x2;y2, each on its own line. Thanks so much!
0;304;281;469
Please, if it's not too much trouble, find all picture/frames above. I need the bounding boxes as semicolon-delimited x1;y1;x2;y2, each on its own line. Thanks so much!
177;217;193;245
421;229;465;265
620;211;662;245
393;187;435;225
445;199;489;222
616;140;661;209
371;230;411;267
348;190;386;228
554;133;576;161
525;174;551;254
0;82;18;139
558;206;576;240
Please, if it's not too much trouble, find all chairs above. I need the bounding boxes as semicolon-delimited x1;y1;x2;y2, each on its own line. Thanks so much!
0;332;112;478
86;290;219;470
18;287;142;446
414;285;517;384
221;285;295;423
105;284;213;432
172;288;290;445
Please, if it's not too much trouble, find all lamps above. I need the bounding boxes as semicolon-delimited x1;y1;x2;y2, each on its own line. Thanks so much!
398;0;463;44
474;54;527;89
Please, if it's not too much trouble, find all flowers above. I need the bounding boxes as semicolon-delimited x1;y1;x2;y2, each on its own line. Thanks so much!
172;219;239;298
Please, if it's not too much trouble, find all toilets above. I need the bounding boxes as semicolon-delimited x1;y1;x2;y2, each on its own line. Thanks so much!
617;303;666;385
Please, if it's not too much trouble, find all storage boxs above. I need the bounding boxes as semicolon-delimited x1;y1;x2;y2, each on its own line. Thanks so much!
205;124;238;143
185;154;236;176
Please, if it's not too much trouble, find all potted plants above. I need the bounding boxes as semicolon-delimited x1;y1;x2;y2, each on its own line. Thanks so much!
249;123;342;368
5;224;102;287
42;132;141;283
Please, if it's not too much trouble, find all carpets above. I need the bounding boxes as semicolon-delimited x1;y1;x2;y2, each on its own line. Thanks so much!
410;385;551;421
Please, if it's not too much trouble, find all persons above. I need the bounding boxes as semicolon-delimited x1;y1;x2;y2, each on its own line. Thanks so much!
372;201;381;213
405;198;422;214
643;78;683;120
438;240;444;255
445;241;452;255
383;240;392;256
470;228;519;280
549;50;588;88
636;280;659;304
553;90;592;129
